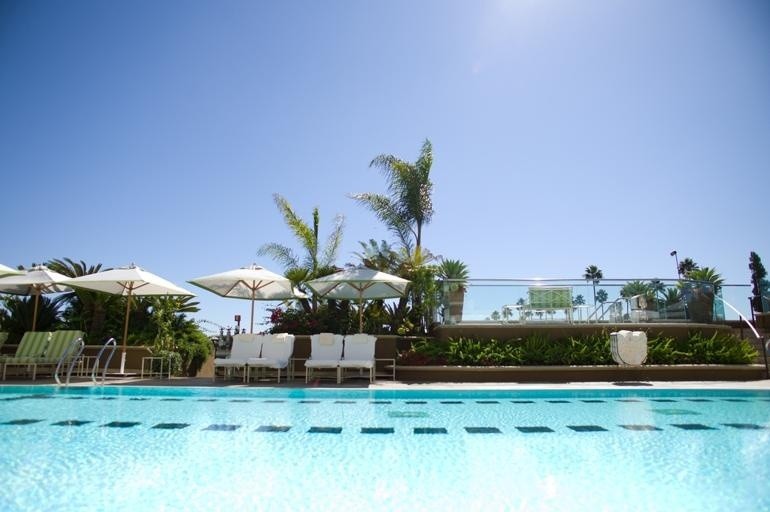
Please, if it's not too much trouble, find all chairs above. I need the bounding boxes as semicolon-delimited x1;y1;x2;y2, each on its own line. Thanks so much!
247;332;297;384
212;331;263;383
338;333;379;384
0;330;89;381
303;333;346;384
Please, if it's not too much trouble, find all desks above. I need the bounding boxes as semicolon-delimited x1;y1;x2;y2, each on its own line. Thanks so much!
139;355;171;380
76;355;99;379
372;358;396;382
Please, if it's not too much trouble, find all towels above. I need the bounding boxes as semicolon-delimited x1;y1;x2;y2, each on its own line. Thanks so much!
354;333;369;344
320;332;334;344
239;333;254;342
272;333;288;347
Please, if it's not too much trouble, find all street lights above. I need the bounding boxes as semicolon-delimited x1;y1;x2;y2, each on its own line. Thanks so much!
669;249;690;321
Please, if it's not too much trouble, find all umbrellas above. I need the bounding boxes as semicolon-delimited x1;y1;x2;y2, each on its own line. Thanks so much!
303;263;413;377
0;263;28;276
186;263;310;334
51;261;198;373
0;266;76;375
